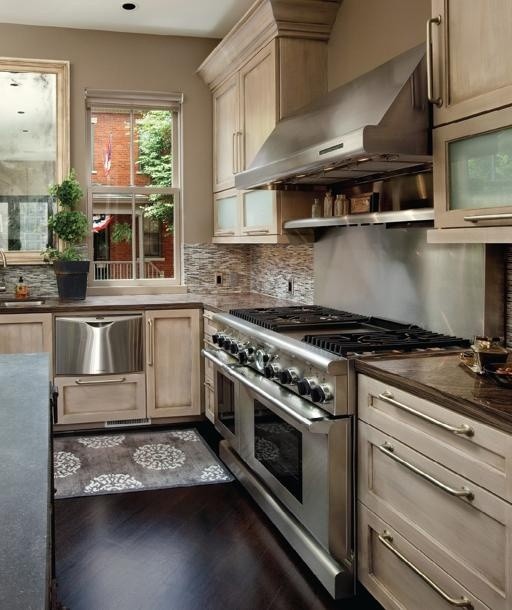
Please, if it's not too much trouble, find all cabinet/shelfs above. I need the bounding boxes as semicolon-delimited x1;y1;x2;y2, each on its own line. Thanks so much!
424;0;512;243
211;186;318;245
353;376;510;609
52;372;147;426
194;0;342;194
144;311;202;420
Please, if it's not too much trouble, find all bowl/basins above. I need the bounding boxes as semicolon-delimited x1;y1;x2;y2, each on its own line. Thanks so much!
483;363;512;387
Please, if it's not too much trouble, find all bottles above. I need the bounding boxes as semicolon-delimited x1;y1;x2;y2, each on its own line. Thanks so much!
311;191;350;218
15;276;27;300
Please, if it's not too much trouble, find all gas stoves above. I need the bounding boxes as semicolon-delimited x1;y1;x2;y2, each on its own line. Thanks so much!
211;305;473;415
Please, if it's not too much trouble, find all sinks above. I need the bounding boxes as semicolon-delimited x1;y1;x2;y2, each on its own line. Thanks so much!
0;297;45;306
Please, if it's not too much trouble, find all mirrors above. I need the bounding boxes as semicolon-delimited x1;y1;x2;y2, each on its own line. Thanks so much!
2;54;73;267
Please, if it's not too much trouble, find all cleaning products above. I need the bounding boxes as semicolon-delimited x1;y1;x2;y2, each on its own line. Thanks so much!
16;276;27;295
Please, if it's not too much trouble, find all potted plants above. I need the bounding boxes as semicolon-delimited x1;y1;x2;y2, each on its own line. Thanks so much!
40;167;93;300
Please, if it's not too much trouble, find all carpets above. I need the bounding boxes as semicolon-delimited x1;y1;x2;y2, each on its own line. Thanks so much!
54;427;237;500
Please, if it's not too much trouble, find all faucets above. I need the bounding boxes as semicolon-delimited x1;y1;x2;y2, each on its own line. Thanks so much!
0;247;7;290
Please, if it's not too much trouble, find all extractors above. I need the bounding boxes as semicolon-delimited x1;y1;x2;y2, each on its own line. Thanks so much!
235;40;432;191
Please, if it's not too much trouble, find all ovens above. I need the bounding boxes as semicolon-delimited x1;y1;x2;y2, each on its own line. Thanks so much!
201;349;352;560
